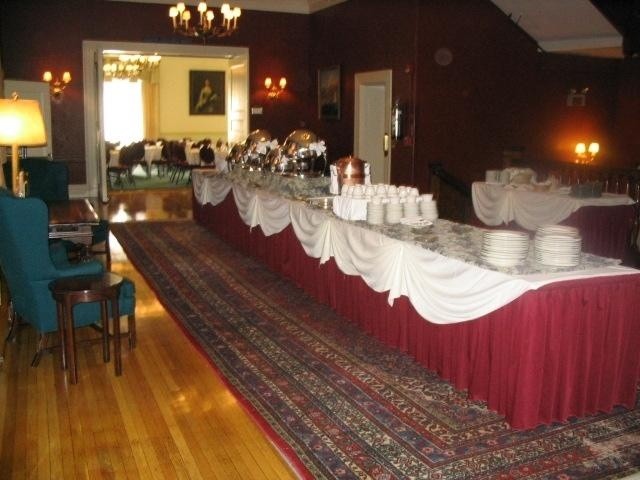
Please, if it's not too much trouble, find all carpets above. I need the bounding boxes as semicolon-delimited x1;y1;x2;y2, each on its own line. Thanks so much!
106;219;640;480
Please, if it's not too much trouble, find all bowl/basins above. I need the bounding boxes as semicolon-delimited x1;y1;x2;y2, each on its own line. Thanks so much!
533;183;553;192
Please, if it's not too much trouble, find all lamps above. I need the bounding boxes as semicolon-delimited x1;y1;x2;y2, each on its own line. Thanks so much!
264;78;287;101
0;99;48;200
169;2;241;48
102;51;162;84
43;71;73;99
575;143;601;165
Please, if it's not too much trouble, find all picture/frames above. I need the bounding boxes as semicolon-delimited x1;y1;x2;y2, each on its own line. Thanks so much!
318;66;340;120
189;69;225;115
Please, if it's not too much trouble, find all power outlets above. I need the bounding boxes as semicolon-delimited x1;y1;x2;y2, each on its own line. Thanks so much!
252;107;262;115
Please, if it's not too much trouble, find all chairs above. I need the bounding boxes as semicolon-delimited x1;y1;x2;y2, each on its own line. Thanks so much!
104;135;227;192
0;198;137;368
0;154;69;202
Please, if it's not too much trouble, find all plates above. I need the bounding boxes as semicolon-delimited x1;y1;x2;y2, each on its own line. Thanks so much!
479;230;528;269
485;170;510;185
385;204;402;224
404;204;419;217
367;202;384;226
420;201;439;220
535;225;582;267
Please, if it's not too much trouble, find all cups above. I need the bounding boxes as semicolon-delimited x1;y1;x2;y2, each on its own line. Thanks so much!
341;183;434;204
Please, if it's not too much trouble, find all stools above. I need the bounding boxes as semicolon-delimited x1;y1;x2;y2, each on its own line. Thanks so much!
66;219;111;265
48;271;124;385
